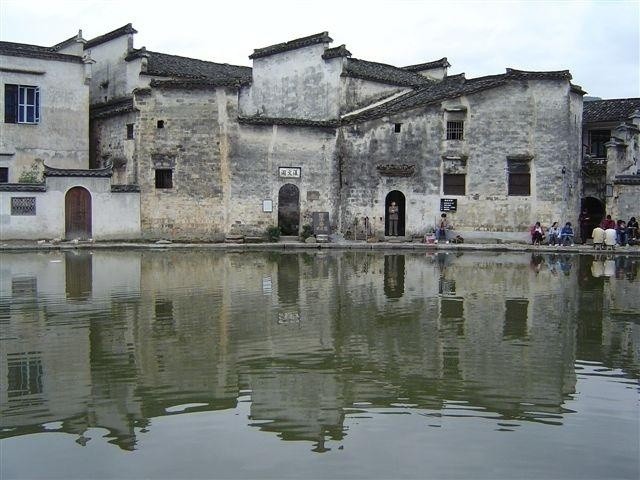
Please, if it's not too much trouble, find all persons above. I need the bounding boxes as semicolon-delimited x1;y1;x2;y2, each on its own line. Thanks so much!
548;221;561;247
615;219;630;248
580;207;589;244
386;255;399;294
437;252;460;285
628;216;638;229
527;252;638;283
388;198;399;236
560;222;575;247
435;213;450;245
591;225;604;248
600;215;615;230
604;226;617;251
531;221;546;246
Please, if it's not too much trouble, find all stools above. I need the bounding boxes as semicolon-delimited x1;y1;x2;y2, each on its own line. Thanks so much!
606;245;613;250
534;240;540;246
606;255;614;260
594;254;603;261
595;243;602;250
